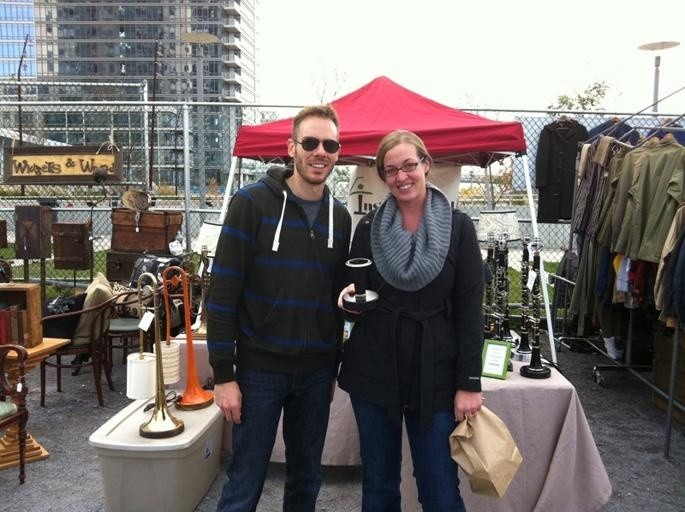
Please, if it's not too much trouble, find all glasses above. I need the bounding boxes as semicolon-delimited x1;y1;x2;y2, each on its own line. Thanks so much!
295;138;341;153
382;157;425;176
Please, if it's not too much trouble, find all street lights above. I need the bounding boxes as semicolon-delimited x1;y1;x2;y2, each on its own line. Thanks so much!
178;29;220;240
636;41;680;116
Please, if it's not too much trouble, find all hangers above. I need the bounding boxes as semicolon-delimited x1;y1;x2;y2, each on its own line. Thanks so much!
555;113;681;153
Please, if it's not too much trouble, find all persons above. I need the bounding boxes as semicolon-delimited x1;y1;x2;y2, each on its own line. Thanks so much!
206;106;352;512
333;130;485;512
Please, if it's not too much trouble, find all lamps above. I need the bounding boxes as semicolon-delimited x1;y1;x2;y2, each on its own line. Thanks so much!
190;220;223;259
478;205;523;265
150;264;215;412
123;271;187;439
83;163;109;288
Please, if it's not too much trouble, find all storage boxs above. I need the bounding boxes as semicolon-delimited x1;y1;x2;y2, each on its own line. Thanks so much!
87;388;227;512
103;249;141;282
12;205;52;259
109;208;184;256
49;218;93;270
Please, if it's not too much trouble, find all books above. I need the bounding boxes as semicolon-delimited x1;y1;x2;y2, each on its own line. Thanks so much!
0;301;31;349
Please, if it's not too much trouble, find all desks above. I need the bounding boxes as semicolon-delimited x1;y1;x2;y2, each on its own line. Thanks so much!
1;336;74;469
394;357;615;512
177;334;385;466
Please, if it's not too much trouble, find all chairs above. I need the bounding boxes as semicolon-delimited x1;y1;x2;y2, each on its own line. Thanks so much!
93;280;172;365
2;343;35;486
36;286;121;408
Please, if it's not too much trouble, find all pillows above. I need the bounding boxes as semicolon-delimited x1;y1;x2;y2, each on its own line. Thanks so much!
110;281;152;318
73;271;116;344
41;292;87;343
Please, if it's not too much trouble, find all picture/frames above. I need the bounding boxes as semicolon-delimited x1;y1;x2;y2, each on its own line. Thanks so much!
1;145;126;186
480;339;513;379
